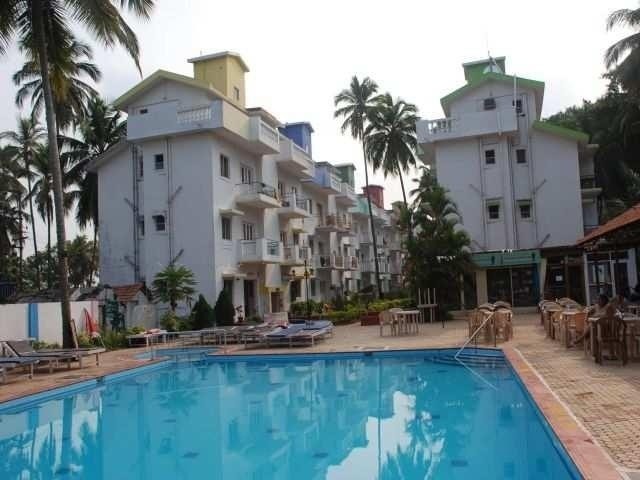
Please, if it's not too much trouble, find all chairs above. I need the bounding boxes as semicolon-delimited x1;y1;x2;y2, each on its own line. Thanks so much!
464;300;513;347
538;297;640;366
0;340;107;384
378;308;405;336
124;320;335;360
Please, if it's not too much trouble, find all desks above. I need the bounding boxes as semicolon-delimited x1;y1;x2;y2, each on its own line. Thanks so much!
395;288;438;337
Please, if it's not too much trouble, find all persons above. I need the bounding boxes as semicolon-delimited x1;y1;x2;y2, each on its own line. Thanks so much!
566;292;622;348
